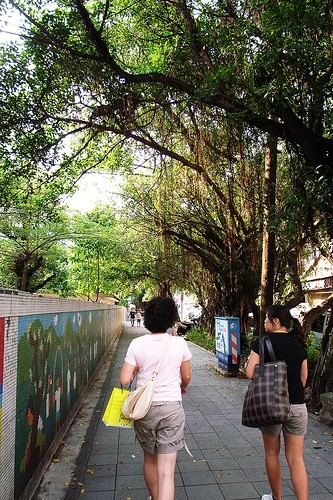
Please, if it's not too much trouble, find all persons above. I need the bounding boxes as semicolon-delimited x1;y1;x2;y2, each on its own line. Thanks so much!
118;297;192;500
128;307;136;327
244;305;308;500
135;311;141;327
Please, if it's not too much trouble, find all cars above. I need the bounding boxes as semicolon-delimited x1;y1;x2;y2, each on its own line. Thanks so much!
310;310;331;348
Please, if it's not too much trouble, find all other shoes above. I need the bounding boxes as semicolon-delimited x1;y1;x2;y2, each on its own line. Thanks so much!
262;493;273;500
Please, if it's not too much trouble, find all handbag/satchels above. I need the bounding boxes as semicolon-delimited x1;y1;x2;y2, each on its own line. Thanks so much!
121;333;172;420
241;336;291;428
101;378;135;429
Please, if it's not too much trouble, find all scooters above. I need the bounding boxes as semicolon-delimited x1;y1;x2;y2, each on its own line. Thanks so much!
177;314;199;336
